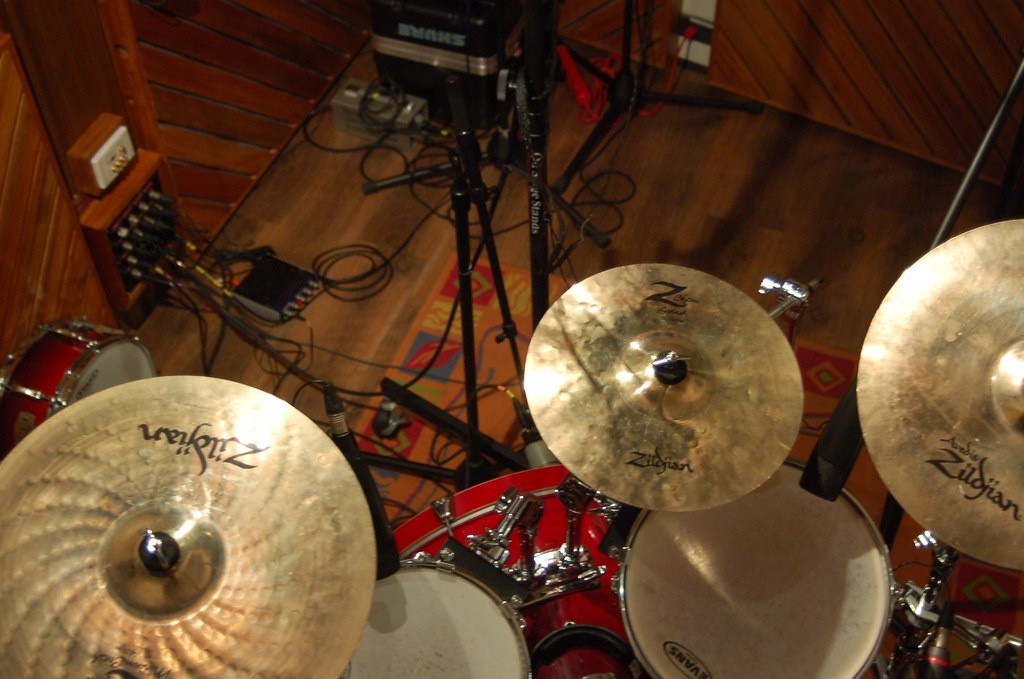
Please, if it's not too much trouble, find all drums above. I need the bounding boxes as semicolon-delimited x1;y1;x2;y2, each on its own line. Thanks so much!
610;456;900;679
389;462;651;679
339;553;532;679
0;317;154;459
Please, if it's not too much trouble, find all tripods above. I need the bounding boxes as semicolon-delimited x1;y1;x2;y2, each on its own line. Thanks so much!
358;0;765;499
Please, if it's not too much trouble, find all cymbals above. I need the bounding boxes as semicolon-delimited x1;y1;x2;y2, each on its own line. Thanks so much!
856;218;1023;574
3;375;376;678
522;262;805;512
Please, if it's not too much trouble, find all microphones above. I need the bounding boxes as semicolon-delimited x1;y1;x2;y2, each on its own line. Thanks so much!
918;602;959;679
